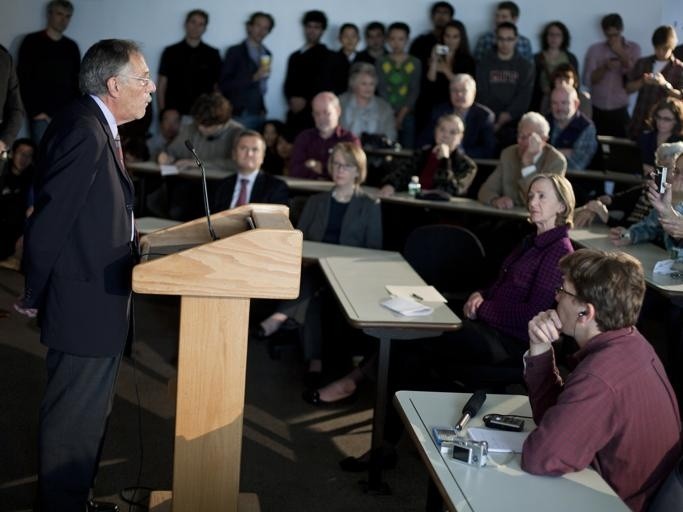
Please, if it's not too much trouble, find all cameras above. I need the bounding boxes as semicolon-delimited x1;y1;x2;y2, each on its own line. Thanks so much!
436;45;449;54
451;437;487;467
653;166;670;194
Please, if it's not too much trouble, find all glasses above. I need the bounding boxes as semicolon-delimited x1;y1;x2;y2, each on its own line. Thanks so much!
332;163;353;171
654;115;673;121
121;74;149;85
553;284;579;299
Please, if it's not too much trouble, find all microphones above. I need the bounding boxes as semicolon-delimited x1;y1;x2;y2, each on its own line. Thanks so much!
451;387;490;430
184;137;218;242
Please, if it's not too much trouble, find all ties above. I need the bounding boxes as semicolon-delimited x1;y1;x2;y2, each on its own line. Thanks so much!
115;134;125;169
235;179;249;207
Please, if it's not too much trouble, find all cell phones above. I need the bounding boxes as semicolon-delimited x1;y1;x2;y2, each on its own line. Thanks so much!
483;412;528;432
433;427;458;445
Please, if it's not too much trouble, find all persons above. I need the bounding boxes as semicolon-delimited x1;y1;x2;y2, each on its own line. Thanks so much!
0;137;36;261
298;173;574;471
14;38;157;512
248;143;382;377
520;248;683;512
116;0;682;260
0;44;24;173
15;1;83;160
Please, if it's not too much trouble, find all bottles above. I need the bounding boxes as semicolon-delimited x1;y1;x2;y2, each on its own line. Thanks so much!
408;176;421;198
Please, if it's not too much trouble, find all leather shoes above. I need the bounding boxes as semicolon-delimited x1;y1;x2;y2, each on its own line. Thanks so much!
256;317;280;339
87;495;119;511
302;385;367;408
304;361;324;380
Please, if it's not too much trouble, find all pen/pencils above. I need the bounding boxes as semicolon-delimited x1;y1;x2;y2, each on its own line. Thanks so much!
413;293;423;300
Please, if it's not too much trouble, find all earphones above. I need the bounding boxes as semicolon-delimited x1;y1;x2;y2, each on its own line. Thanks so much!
579;309;587;319
